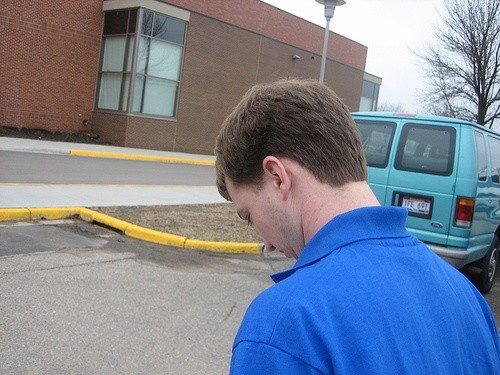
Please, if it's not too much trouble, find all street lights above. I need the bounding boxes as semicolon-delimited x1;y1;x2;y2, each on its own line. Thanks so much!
315;0;346;85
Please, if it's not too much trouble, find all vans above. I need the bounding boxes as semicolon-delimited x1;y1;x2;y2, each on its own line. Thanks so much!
347;112;500;294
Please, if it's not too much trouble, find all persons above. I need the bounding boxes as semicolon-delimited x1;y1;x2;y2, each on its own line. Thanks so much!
214;78;500;375
